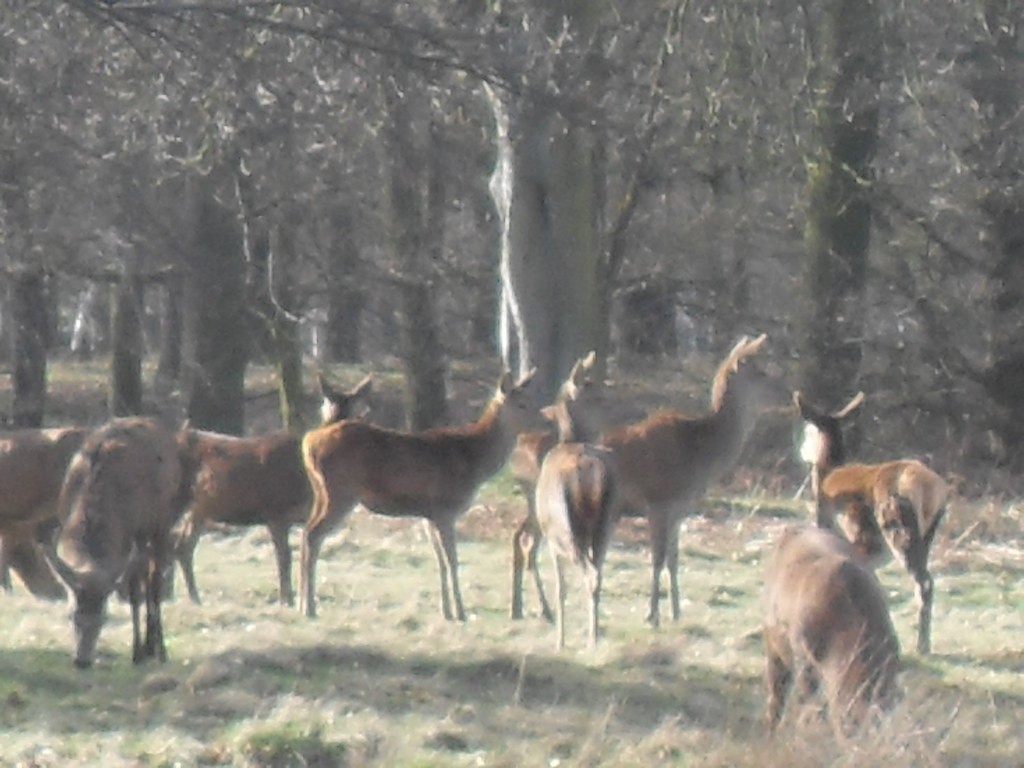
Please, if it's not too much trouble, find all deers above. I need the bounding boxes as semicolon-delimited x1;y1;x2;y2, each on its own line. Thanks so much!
761;387;950;756
511;332;797;652
1;365;554;674
